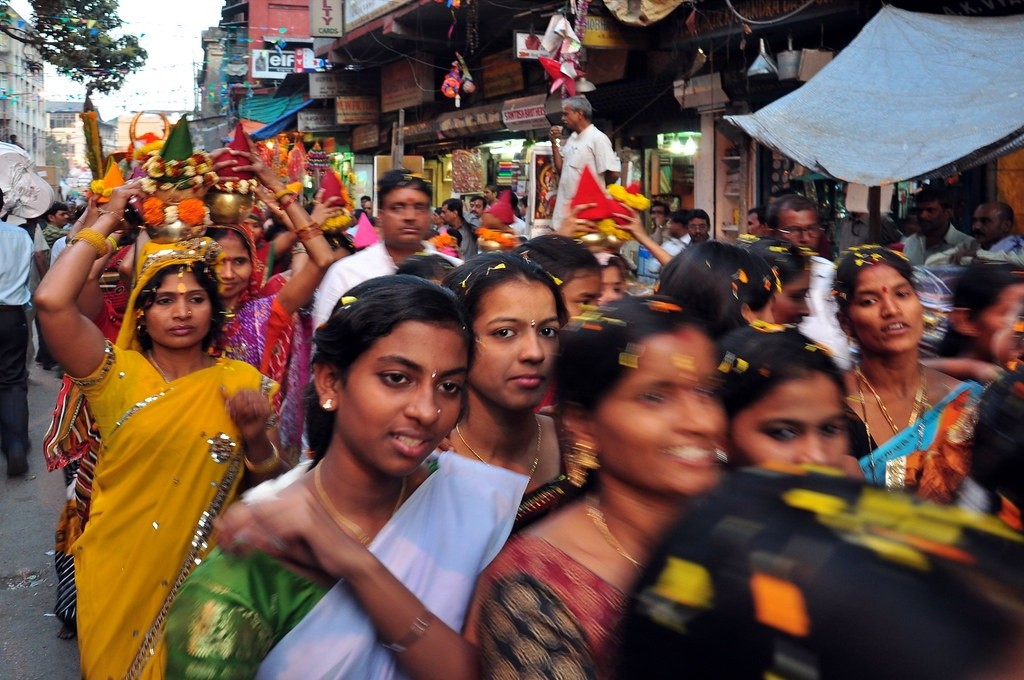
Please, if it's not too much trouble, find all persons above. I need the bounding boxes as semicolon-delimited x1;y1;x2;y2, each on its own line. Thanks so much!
0;95;1023;678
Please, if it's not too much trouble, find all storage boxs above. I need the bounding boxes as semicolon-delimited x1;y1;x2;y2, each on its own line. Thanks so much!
35;164;61;201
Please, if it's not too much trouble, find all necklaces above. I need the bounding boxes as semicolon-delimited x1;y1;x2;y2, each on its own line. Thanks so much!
854;373;878;486
146;349;169;382
853;362;927;437
314;458;407;546
456;413;541;478
586;492;645;577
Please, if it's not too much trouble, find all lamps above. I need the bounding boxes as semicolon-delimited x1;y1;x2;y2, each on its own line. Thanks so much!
669;134;683;154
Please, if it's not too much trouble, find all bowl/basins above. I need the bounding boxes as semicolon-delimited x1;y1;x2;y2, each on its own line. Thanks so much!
144;198;210;244
480;228;513;252
579;229;624;252
205;188;255;224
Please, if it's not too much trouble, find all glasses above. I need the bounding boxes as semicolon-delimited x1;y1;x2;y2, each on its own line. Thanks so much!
777;226;824;239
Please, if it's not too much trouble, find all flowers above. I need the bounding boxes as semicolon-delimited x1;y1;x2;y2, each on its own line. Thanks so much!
140;151;219;194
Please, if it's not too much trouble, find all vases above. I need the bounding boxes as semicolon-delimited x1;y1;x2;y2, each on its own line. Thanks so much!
156;180;210;203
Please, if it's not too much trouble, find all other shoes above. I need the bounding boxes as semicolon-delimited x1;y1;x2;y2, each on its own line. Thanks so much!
6;445;28;477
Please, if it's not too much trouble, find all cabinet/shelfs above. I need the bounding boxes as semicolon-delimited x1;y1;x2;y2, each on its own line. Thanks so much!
713;118;753;240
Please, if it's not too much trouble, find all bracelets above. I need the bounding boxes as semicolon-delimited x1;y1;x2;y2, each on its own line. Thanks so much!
77;218;86;225
71;228;109;260
294;220;319;236
300;231;323;242
277;192;298;210
378;610;433;652
273;190;295;202
245;442;279;474
290;247;306;254
107;235;118;252
97;208;128;233
